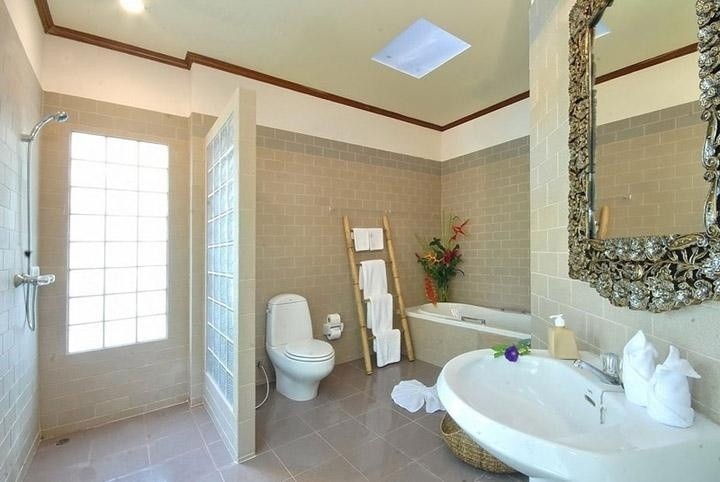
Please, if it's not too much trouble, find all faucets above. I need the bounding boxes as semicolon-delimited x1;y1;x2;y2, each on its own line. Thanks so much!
573;351;620;386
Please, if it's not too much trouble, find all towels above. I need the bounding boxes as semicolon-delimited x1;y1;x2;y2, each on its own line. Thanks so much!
391;378;446;413
367;293;393;336
359;259;388;300
373;329;401;368
350;227;369;252
648;345;701;428
621;330;658;407
368;228;384;251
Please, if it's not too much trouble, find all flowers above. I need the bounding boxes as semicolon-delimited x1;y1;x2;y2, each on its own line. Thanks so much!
491;343;531;362
414;214;470;306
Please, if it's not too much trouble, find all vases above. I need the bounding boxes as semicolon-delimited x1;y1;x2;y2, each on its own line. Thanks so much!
438;280;448;301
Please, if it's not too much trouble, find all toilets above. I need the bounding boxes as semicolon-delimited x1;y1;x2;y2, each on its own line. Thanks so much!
264;293;335;401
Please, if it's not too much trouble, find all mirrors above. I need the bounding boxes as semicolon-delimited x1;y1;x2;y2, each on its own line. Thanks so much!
566;0;720;312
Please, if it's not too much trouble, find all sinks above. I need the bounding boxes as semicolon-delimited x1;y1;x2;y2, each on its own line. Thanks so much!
443;348;720;482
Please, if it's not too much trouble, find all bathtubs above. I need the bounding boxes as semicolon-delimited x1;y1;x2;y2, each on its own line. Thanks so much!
396;299;532;367
